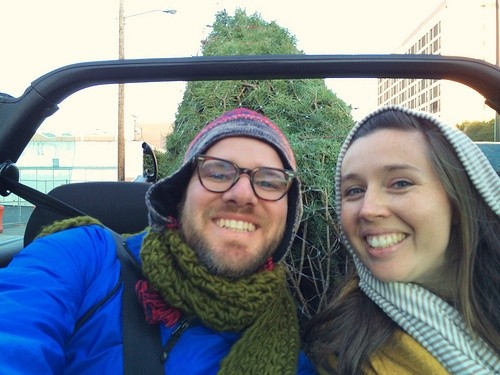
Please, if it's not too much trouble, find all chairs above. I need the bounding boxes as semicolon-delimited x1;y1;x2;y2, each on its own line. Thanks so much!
22;180;153;252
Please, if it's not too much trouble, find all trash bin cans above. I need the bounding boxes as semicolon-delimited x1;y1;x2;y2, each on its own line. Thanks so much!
0;205;4;233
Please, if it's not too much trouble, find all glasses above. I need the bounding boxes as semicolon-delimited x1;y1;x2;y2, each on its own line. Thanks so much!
194;155;295;202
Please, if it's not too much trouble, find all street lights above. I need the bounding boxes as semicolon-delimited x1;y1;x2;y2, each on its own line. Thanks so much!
117;1;178;181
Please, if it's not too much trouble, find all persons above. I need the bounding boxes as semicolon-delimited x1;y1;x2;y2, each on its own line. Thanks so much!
1;107;320;374
299;104;498;374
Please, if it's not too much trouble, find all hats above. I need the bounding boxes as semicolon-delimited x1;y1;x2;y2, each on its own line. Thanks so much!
146;109;304;272
